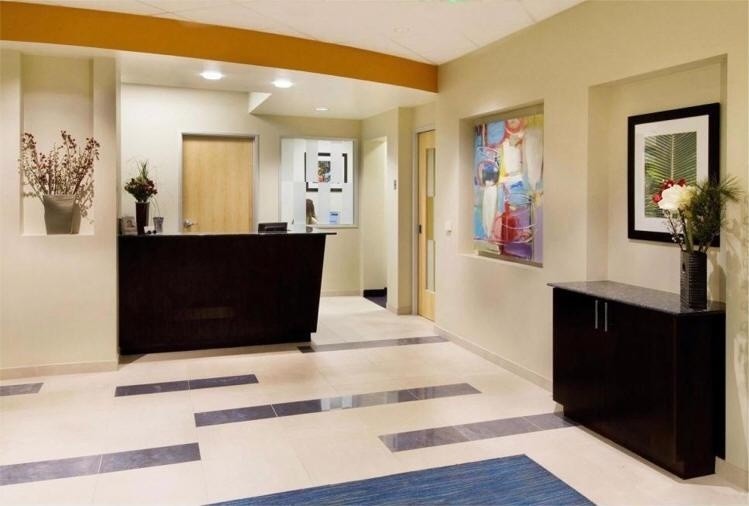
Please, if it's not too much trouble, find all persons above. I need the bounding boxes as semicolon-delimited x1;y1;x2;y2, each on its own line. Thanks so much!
306;199;318;224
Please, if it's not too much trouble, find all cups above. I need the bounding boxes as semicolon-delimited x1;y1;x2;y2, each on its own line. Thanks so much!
473;145;500;187
153;217;164;233
503;192;533;249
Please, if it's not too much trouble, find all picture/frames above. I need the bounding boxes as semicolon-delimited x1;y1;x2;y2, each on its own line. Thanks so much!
628;102;721;247
305;152;347;191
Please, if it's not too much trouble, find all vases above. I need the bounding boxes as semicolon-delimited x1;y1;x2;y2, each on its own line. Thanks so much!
136;201;149;234
680;250;707;310
43;195;80;234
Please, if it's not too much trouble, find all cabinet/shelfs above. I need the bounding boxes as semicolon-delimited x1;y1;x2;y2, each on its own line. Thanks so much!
546;280;726;480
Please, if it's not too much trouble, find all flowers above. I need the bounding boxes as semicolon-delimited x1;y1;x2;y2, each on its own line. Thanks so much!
16;128;99;204
125;159;161;217
651;172;745;252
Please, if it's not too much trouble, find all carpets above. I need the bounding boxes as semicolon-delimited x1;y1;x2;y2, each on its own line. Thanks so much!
197;453;599;506
